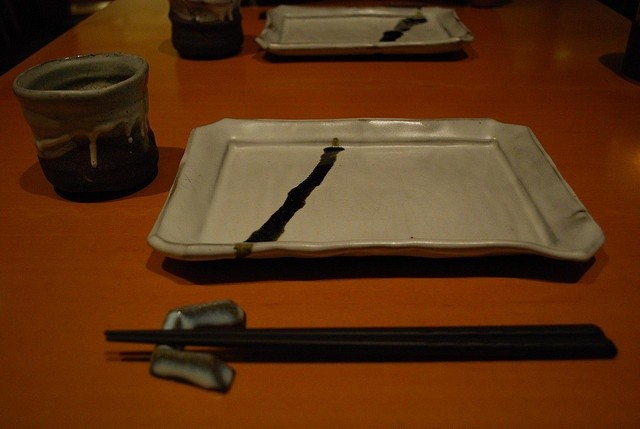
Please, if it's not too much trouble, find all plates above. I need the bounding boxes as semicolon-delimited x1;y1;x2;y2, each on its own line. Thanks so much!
254;4;475;56
144;117;607;262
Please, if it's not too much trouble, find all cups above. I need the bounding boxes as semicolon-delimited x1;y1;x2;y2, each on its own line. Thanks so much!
168;1;245;61
10;52;160;203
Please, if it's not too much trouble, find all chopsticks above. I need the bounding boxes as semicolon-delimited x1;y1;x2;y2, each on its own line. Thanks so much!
103;323;606;338
102;335;619;363
